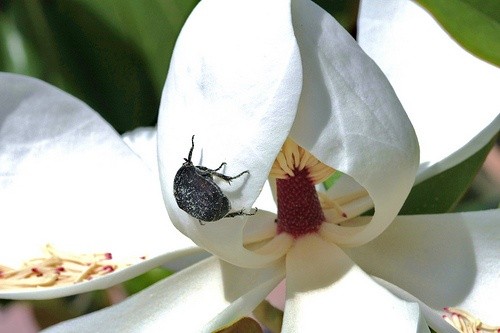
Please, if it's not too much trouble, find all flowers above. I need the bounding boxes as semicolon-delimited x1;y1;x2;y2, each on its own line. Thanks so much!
0;0;500;332
